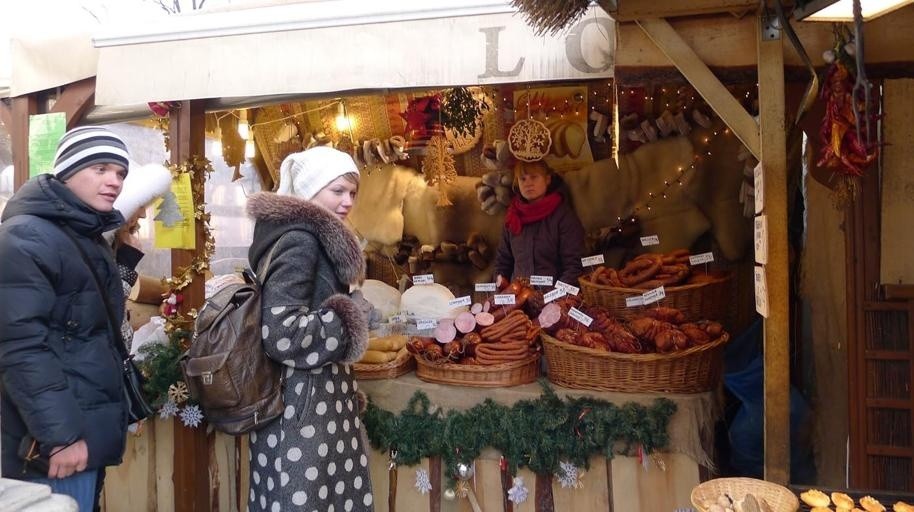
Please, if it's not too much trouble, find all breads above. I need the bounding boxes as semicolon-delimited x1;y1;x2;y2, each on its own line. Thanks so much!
702;493;774;512
540;117;586;159
800;488;914;512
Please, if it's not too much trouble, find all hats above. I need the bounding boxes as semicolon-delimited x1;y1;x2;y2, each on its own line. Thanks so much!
54;125;130;182
277;145;360;200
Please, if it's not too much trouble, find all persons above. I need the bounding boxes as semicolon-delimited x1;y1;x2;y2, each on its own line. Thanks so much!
247;145;377;511
488;158;590;297
104;161;174;362
0;125;129;511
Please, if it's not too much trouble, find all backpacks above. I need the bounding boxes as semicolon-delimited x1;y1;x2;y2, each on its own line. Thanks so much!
179;233;288;436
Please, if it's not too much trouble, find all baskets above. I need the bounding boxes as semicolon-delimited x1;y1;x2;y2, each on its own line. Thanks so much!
415;344;544;388
365;269;413;291
353;353;414;382
691;476;801;512
353;270;736;394
579;273;733;322
442;281;499;306
540;310;729;395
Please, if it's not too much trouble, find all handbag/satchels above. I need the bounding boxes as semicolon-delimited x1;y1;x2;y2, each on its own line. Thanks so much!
120;357;155;425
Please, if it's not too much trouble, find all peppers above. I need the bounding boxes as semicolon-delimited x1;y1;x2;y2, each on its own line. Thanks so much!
815;39;894;179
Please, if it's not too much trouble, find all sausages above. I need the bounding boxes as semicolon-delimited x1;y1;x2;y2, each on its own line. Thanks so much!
588;248;693;290
357;274;532;367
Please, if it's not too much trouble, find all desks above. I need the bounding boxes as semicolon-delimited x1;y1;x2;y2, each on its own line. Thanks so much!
352;357;725;511
